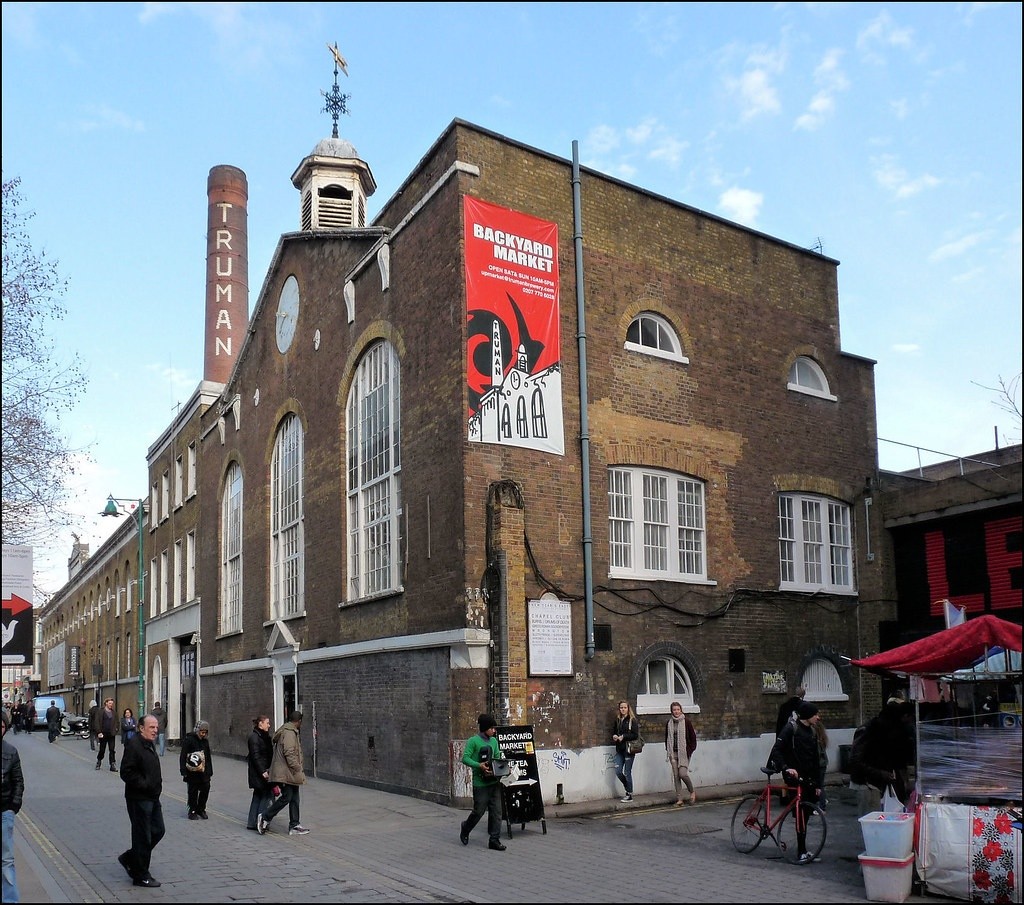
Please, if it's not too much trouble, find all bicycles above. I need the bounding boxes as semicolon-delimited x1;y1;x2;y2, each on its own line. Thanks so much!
730;766;828;865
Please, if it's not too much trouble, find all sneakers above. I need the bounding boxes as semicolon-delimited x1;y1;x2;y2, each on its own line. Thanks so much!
118;852;133;878
805;851;822;862
489;840;506;850
289;824;310;835
133;875;161;887
460;820;468;845
797;852;810;862
621;792;633;803
257;812;268;835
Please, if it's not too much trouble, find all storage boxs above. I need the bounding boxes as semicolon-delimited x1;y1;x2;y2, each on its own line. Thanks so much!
858;810;917;858
858;850;914;903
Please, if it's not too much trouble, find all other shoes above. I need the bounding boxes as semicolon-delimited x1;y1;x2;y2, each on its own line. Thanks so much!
96;760;101;770
110;763;118;772
196;808;208;819
188;807;197;819
673;800;684;807
689;791;695;806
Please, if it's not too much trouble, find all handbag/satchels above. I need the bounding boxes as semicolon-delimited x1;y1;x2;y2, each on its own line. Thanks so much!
880;783;907;814
185;750;205;772
626;739;642;753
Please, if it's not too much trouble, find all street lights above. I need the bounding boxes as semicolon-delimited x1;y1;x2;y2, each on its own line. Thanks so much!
97;493;145;726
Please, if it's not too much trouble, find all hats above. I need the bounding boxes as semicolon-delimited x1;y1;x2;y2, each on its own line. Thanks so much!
798;702;819;719
194;720;209;740
886;690;905;705
2;707;8;736
478;714;497;732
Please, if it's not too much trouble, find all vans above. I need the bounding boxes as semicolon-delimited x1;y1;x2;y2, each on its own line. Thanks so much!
29;697;66;731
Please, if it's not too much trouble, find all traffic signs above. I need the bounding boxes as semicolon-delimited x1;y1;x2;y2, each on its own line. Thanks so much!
500;728;545;825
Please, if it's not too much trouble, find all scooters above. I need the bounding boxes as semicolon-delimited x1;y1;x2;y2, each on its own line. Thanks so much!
55;713;91;740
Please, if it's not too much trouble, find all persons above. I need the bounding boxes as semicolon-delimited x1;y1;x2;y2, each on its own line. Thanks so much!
118;713;165;887
612;701;644;803
0;698;37;736
664;702;697;808
848;700;916;821
247;711;310;837
46;701;61;743
151;701;167;757
87;697;118;773
460;714;507;852
180;719;213;820
2;711;25;903
121;709;137;746
763;686;829;865
